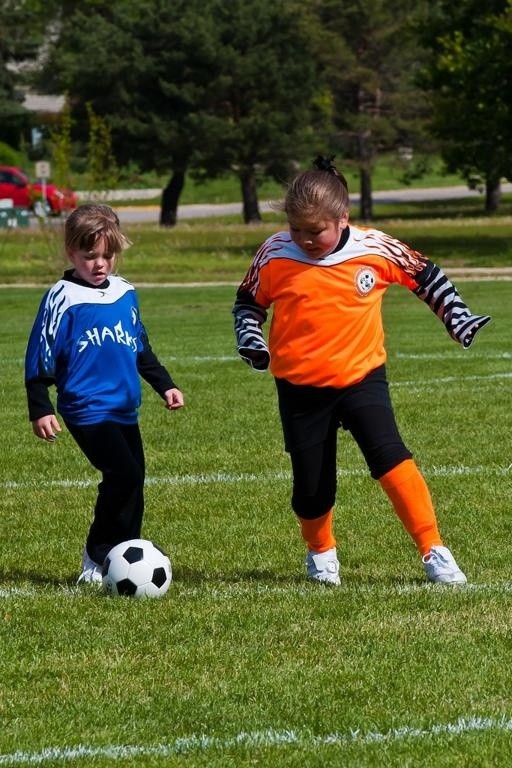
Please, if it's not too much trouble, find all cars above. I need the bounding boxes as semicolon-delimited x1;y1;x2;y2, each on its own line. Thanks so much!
0;166;77;219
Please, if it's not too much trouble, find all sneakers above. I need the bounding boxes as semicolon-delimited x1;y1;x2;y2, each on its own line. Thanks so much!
307;547;343;587
421;544;467;587
76;548;103;589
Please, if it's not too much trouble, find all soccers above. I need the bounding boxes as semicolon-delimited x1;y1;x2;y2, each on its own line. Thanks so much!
102;538;172;599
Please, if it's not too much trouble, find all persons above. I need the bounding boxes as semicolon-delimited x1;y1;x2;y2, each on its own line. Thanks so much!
232;162;491;586
25;204;185;582
